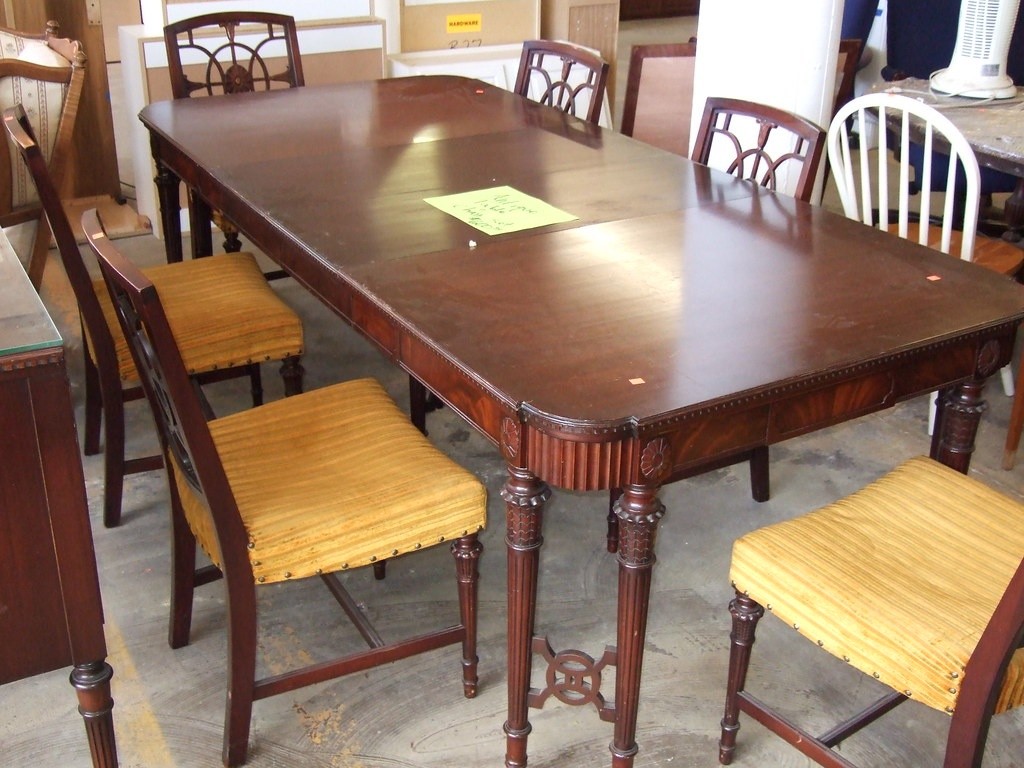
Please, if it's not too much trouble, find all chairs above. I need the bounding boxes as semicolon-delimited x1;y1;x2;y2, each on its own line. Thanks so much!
513;38;611;127
607;96;828;553
4;104;305;528
163;11;305;253
721;457;1024;767
827;93;1024;437
0;23;87;294
78;210;488;768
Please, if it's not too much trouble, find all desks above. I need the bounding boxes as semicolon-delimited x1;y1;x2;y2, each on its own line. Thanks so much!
137;74;1024;768
856;64;1024;245
